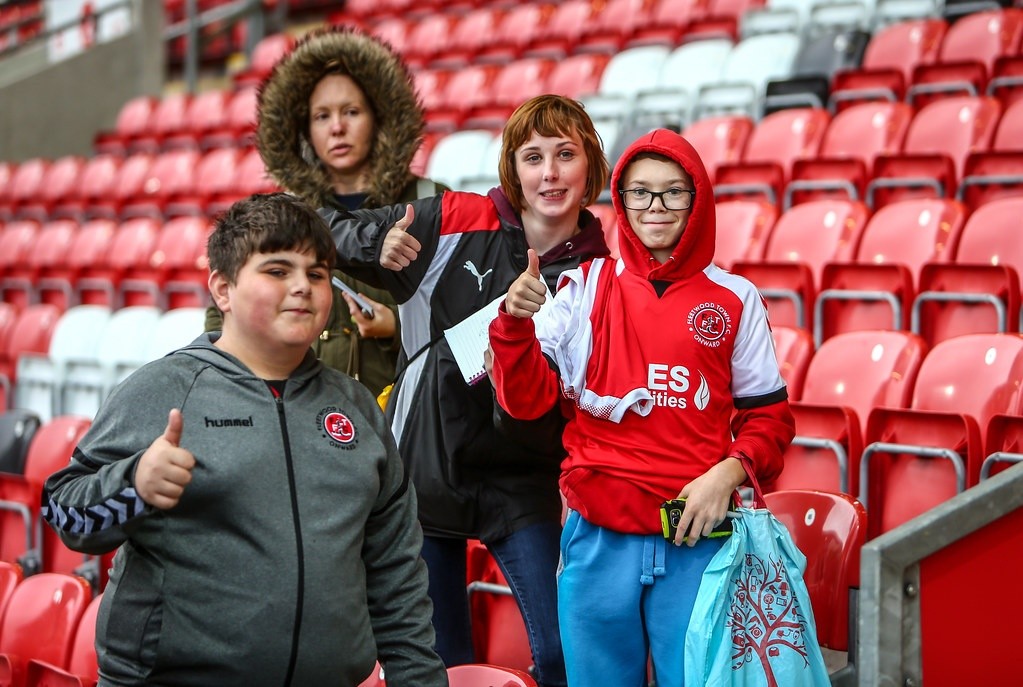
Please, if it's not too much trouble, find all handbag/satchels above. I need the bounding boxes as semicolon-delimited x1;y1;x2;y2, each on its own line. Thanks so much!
683;454;831;687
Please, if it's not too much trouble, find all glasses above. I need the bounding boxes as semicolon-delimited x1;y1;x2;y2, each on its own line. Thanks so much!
617;188;695;210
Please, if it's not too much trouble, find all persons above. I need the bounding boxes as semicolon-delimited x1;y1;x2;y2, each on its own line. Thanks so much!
40;192;449;687
205;26;452;395
315;95;611;687
490;128;796;687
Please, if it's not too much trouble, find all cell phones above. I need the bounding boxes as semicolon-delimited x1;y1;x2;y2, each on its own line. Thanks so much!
330;276;375;318
659;496;735;543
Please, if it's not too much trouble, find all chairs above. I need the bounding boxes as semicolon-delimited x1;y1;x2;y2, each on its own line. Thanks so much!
0;1;1023;687
